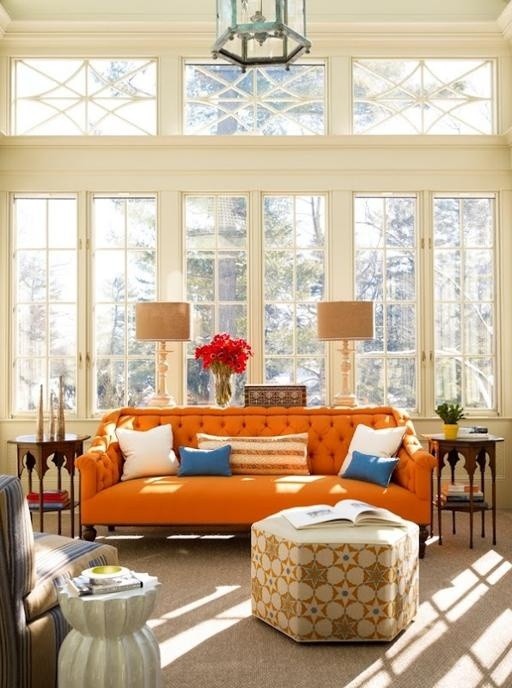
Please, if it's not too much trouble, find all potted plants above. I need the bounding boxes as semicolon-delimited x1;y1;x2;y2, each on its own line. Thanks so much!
432;402;467;442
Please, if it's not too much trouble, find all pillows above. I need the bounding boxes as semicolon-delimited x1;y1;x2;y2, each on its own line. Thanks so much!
337;420;406;484
115;423;181;480
188;431;311;478
342;451;400;487
173;443;234;478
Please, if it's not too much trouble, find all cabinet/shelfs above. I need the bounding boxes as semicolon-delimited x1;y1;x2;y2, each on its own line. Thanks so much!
6;432;92;536
417;427;505;551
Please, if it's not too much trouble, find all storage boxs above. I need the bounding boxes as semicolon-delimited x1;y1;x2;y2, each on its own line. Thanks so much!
244;384;308;407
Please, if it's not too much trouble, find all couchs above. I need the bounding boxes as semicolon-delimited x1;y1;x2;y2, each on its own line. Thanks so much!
77;403;441;557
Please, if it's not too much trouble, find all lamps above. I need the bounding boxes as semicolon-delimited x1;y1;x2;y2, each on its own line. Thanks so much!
134;301;193;408
205;0;314;70
316;302;374;405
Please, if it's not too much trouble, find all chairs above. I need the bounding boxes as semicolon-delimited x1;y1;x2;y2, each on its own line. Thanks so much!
1;475;124;687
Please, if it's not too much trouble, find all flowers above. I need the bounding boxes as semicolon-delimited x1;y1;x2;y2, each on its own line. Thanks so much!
197;332;254;407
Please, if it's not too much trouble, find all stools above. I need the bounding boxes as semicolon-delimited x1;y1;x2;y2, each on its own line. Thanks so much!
248;512;421;647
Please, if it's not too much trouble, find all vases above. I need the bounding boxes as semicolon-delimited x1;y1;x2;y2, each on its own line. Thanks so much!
211;372;236;407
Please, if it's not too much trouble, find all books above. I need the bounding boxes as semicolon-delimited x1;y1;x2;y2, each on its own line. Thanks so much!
441;484;483;502
455;427;487;439
281;498;405;531
66;575;143;597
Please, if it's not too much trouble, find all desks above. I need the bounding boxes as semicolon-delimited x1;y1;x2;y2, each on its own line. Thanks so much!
57;573;163;688
